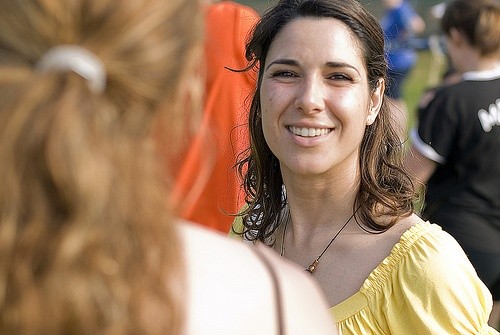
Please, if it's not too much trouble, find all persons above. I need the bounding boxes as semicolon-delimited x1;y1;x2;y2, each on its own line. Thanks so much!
167;0;500;335
0;0;335;335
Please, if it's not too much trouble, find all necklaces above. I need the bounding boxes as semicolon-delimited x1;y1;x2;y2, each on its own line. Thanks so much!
281;204;361;273
